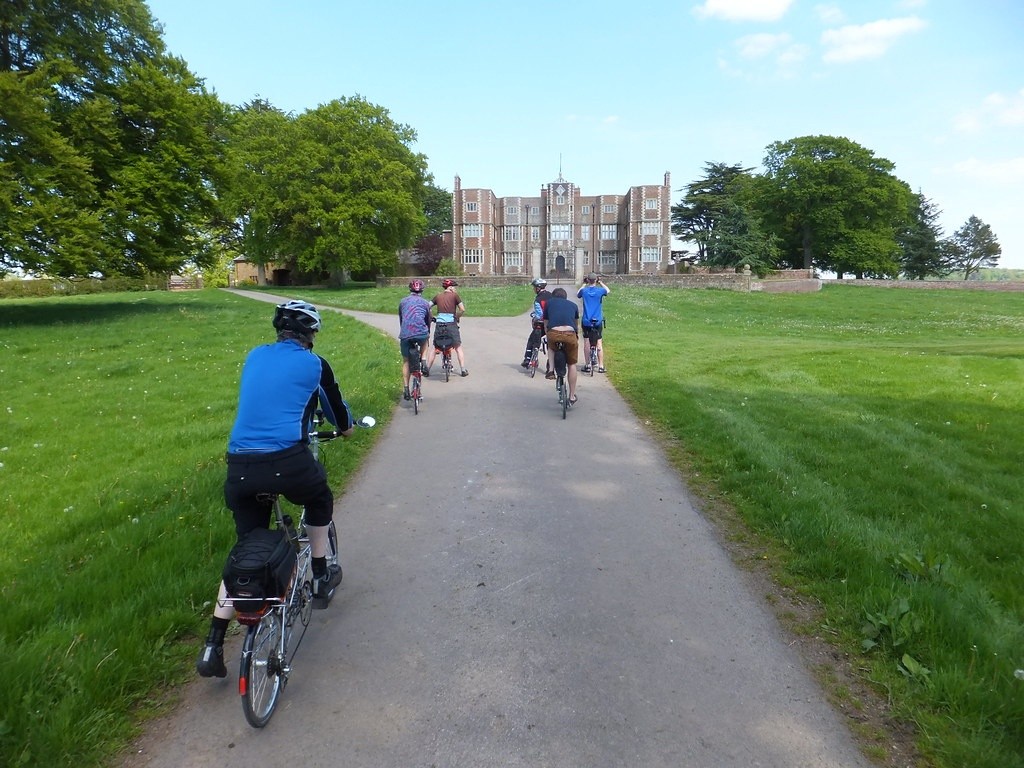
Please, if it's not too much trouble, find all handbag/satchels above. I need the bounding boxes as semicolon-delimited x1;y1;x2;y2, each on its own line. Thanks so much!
223;529;296;611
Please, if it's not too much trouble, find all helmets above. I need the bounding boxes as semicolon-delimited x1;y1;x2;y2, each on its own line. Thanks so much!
409;280;424;292
588;272;596;280
531;278;547;287
442;279;458;287
273;299;321;332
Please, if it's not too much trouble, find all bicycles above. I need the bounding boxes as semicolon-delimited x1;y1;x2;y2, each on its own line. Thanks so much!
589;319;607;377
215;409;376;729
406;340;427;415
526;312;546;378
550;342;572;419
432;317;460;382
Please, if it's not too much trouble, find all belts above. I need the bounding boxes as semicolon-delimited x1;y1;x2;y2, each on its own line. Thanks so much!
550;329;575;334
228;443;307;463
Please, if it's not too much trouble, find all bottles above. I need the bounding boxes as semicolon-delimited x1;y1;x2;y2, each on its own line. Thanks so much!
282;515;301;554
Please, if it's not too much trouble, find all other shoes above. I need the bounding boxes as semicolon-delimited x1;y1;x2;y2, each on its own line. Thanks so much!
462;369;468;376
421;365;428;374
570;394;578;403
545;371;555;378
521;360;529;366
404;392;410;400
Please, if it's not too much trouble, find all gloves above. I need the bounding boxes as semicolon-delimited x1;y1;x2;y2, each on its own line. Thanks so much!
584;277;589;284
597;276;601;283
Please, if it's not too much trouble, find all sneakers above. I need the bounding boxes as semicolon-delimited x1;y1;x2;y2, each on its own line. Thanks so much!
198;643;227;678
311;564;342;598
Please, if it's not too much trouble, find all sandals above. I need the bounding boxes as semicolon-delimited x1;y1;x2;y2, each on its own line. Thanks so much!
599;366;606;373
581;364;591;372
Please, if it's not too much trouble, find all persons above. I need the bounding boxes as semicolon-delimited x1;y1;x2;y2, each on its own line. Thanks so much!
520;278;553;373
398;279;433;401
577;273;611;373
427;279;469;377
543;288;580;405
194;300;354;678
